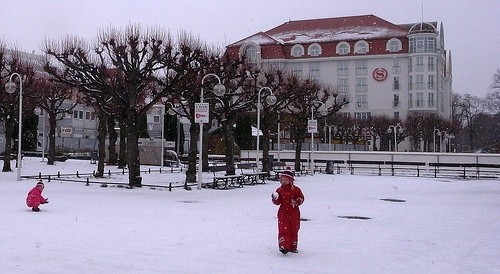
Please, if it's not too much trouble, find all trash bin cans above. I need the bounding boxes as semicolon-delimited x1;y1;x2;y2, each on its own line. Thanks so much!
325;160;335;174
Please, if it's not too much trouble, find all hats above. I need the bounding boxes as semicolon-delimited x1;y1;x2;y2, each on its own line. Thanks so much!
280;170;296;181
36;182;45;189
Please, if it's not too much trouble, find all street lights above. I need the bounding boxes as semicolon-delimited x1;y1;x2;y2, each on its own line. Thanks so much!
311;101;328;176
196;73;226;190
34;106;44;162
256;87;277;173
387;125;403;152
161;102;176;168
5;73;22;181
323;125;337;151
434;129;454;153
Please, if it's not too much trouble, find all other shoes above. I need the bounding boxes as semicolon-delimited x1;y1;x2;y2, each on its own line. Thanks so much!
290;249;299;253
279;246;288;254
32;207;40;212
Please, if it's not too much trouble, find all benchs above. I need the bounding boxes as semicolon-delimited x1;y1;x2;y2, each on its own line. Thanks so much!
208;166;243;190
272;161;296;181
237;163;268;186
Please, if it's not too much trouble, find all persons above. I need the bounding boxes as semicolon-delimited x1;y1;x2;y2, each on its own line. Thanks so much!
26;181;49;211
271;170;305;254
